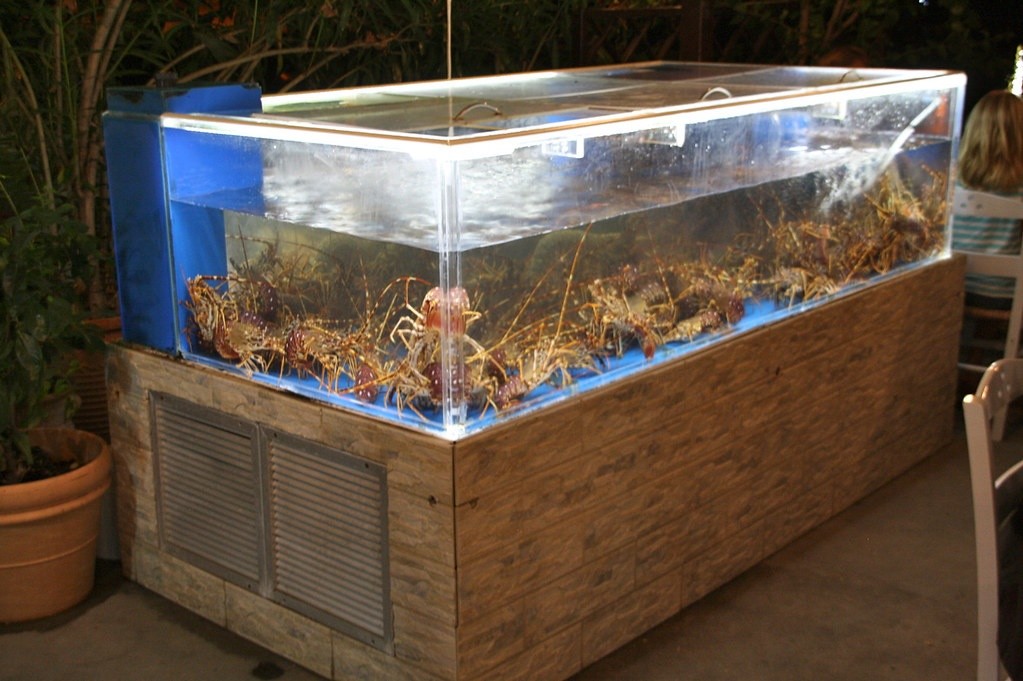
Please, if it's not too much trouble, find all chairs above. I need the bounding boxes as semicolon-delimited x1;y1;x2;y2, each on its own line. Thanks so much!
951;186;1023;440
962;358;1023;681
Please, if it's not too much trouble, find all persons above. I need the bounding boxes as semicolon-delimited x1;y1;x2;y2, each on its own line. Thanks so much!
951;90;1023;426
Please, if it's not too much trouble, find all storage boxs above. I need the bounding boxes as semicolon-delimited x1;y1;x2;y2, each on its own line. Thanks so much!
102;58;967;444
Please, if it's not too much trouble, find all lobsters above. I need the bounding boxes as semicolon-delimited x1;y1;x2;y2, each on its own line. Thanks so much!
178;161;948;425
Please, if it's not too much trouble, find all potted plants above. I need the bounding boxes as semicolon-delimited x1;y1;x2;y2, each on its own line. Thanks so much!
0;1;440;447
0;187;115;622
